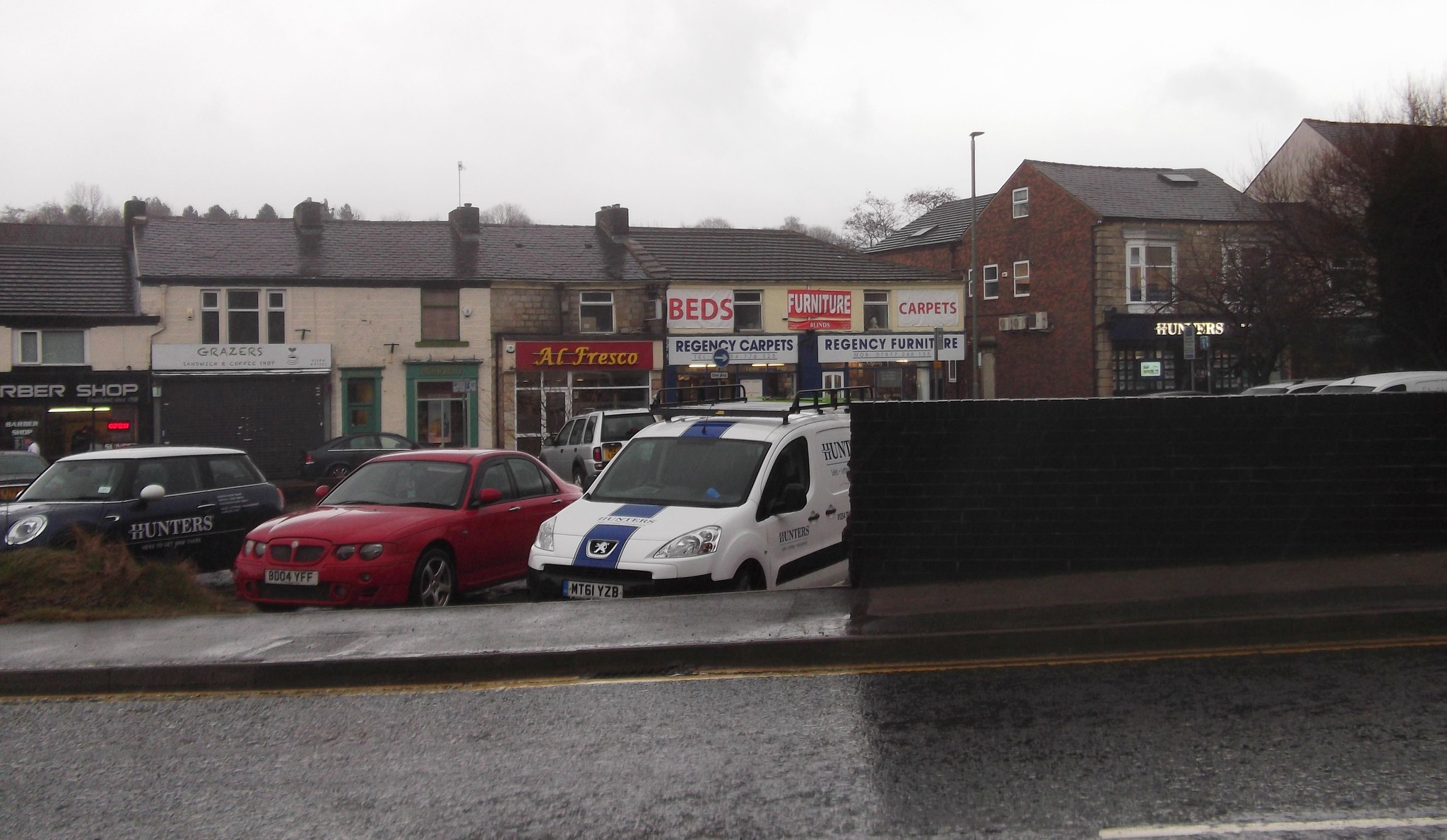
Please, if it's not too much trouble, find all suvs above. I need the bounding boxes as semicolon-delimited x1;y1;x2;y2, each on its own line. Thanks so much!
524;382;874;600
0;444;286;568
538;404;658;488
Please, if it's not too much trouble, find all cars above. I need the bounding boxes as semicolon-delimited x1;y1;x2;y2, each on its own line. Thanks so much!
304;432;423;488
1141;370;1446;396
232;446;584;613
0;450;51;501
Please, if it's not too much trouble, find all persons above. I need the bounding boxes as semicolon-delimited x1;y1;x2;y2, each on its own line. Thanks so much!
69;425;91;453
25;435;40;455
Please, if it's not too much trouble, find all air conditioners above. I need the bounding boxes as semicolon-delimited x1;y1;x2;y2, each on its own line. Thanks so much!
1011;316;1028;330
998;317;1012;331
1029;311;1048;330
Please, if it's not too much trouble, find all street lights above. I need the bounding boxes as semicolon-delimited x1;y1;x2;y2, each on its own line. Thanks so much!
969;131;985;399
